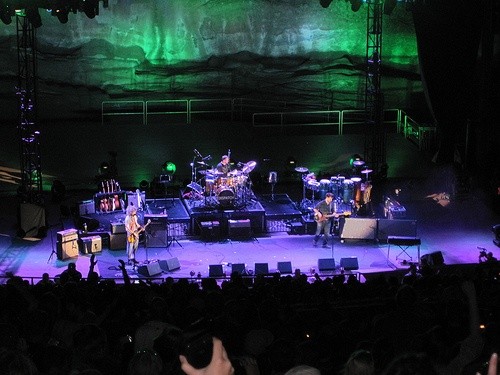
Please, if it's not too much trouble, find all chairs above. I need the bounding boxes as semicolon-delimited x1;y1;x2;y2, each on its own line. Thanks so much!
491;224;500;248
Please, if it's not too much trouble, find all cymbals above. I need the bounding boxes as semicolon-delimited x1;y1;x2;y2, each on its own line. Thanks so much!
361;170;372;173
295;167;309;172
243;160;256;173
228;170;242;174
208;170;223;174
197;161;209;166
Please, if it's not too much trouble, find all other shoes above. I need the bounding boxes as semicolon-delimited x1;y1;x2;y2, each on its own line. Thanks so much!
133;258;139;263
321;244;331;249
312;240;317;248
128;260;132;265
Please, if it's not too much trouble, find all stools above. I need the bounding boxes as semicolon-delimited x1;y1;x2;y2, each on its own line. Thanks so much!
386;236;422;266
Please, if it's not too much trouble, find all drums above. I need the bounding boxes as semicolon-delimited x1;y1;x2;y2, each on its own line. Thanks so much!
217;187;234;205
304;173;361;212
206;175;248;193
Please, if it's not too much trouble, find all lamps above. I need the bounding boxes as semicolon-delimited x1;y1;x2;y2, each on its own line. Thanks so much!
350;0;363;12
320;0;333;8
384;0;397;16
25;7;42;28
52;7;68;24
79;0;99;19
0;0;15;25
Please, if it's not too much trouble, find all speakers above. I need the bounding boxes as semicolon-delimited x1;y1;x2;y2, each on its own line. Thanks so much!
318;258;336;271
183;181;203;194
340;257;358;270
232;263;245;276
144;227;168;248
78;216;100;232
277;261;292;274
125;192;146;209
78;200;95;215
200;221;221;242
341;218;418;243
255;263;268;274
81;235;102;254
137;262;162;277
56;239;78;259
159;257;180;272
209;265;223;277
421;250;444;268
107;232;127;250
228;219;251;241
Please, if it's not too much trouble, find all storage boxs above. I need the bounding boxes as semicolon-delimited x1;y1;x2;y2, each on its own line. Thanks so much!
107;223;126;251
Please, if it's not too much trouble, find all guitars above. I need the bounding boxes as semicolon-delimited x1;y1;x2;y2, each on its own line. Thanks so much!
128;218;151;243
314;210;351;223
100;179;119;211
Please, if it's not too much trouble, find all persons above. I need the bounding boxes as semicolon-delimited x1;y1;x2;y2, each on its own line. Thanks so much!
313;193;339;249
124;206;145;264
0;251;500;375
217;155;230;172
